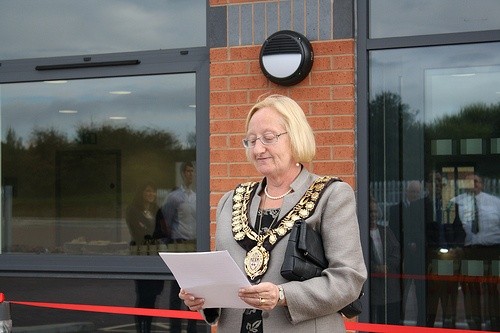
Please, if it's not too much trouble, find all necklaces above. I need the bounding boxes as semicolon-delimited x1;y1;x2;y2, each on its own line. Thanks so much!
264;180;294;200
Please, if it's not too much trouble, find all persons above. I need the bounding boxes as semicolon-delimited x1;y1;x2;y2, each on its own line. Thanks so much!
178;94;368;333
125;181;168;333
161;161;196;333
405;169;467;330
449;175;500;333
388;179;429;327
368;196;402;325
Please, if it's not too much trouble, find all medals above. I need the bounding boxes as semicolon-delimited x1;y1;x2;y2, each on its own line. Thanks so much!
243;245;270;281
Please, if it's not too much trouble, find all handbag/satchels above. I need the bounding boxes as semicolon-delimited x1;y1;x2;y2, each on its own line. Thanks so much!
280;218;363;319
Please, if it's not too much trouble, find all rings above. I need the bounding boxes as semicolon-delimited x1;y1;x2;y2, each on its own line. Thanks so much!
258;297;264;305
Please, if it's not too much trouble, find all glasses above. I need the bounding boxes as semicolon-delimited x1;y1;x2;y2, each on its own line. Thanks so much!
242;131;287;147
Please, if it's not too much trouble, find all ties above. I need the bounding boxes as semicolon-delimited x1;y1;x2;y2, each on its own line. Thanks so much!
471;194;480;236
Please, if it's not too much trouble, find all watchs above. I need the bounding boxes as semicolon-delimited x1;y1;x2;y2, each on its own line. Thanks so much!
278;286;285;304
